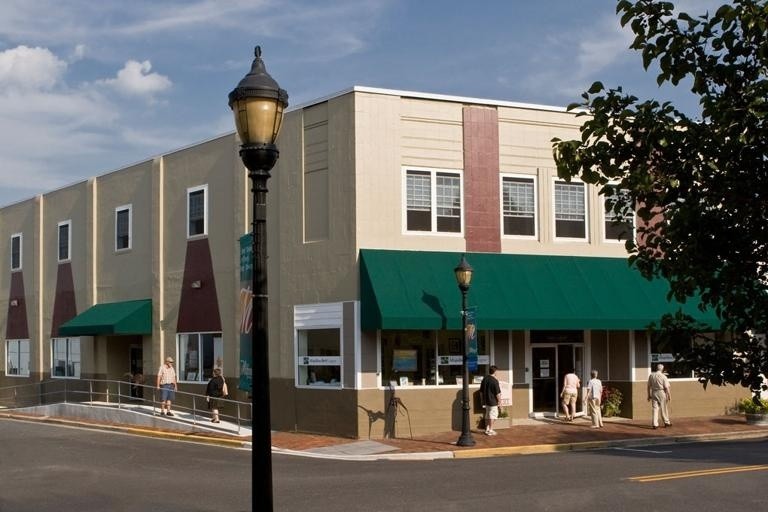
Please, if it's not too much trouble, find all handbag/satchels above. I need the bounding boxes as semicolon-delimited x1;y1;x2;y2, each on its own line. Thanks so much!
222;383;228;396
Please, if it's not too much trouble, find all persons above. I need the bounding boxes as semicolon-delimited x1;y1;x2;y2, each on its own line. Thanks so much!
584;369;604;429
157;356;179;416
646;364;673;430
559;368;581;423
479;366;502;436
205;369;226;425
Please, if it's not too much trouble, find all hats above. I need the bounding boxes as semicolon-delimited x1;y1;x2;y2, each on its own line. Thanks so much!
165;357;174;362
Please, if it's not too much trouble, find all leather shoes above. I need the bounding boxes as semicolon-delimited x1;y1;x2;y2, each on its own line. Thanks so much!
665;423;672;427
654;426;660;428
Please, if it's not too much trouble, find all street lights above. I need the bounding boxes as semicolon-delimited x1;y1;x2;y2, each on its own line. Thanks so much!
227;43;292;511
451;251;475;450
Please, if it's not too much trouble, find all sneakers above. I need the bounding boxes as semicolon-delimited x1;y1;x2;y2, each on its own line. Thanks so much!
161;411;174;416
485;431;497;435
